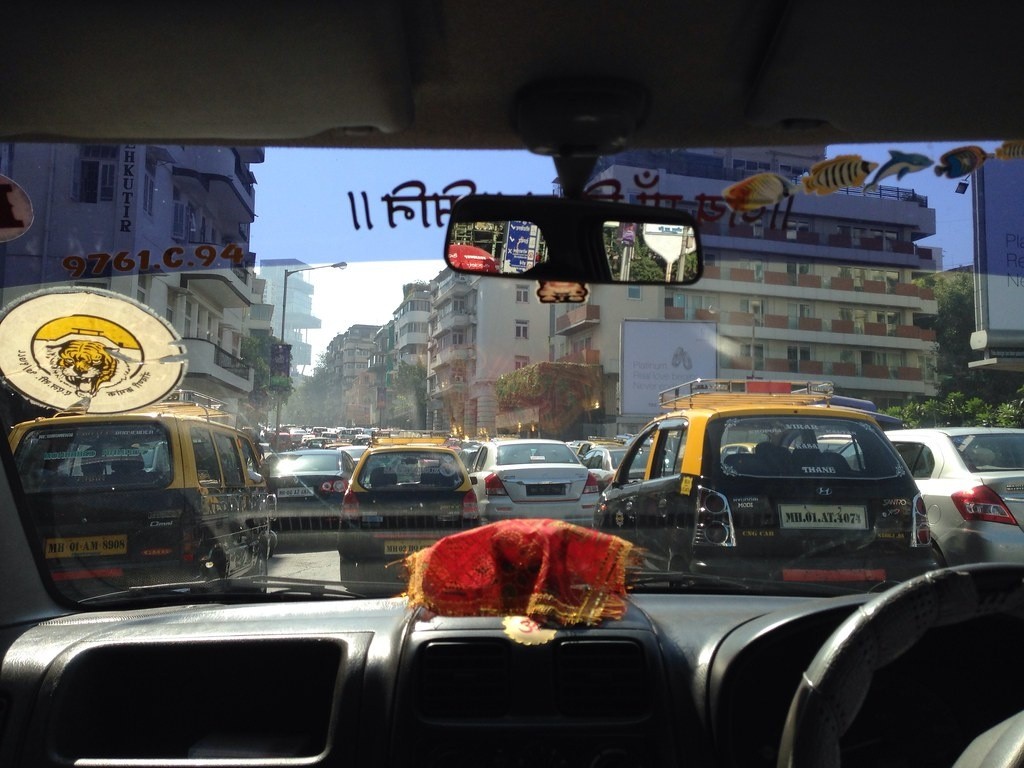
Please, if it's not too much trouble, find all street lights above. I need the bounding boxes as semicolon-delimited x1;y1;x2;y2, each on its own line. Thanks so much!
275;262;347;452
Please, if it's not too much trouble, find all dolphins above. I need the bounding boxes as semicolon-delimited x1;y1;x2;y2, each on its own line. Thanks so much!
862;149;936;194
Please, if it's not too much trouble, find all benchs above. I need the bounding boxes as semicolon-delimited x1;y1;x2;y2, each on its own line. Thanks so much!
725;450;850;476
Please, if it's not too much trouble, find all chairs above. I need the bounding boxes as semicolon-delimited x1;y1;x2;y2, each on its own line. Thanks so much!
369;467;397;488
111;447;147;478
419;465;448;485
81;446;107;477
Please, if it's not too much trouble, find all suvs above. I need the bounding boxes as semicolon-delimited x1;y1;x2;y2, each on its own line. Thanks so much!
838;428;1024;562
591;379;948;598
317;437;482;594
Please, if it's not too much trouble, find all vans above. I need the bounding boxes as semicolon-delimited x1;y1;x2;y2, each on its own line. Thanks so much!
7;390;276;602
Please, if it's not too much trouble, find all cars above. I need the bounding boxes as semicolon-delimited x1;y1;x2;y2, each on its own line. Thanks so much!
262;450;356;503
142;425;855;490
468;439;599;528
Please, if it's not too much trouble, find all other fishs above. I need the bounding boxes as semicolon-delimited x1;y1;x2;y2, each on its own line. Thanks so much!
933;136;1024;179
721;154;879;216
